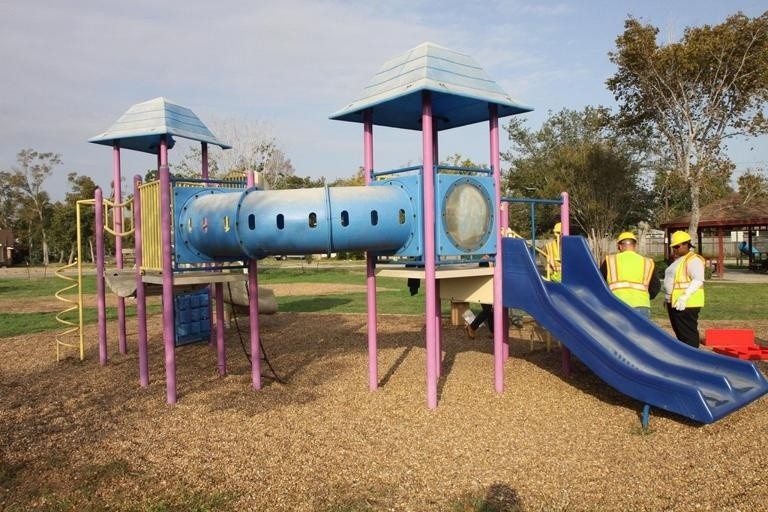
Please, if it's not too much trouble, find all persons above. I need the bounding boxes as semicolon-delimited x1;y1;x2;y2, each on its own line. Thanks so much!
540;222;561;283
663;230;706;348
466;254;496;339
600;232;661;320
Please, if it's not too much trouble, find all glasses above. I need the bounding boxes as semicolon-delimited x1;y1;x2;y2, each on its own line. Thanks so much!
674;245;680;249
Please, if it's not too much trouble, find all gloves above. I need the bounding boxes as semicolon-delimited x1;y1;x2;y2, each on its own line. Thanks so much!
675;295;686;311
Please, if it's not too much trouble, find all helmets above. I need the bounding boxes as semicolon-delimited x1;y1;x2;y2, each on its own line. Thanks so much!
552;223;561;232
669;230;691;247
616;232;638;244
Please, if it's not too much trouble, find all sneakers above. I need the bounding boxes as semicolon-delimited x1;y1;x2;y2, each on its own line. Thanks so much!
466;325;476;338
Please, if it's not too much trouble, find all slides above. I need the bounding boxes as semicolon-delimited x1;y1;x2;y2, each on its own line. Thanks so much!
211;283;278;314
502;236;767;424
